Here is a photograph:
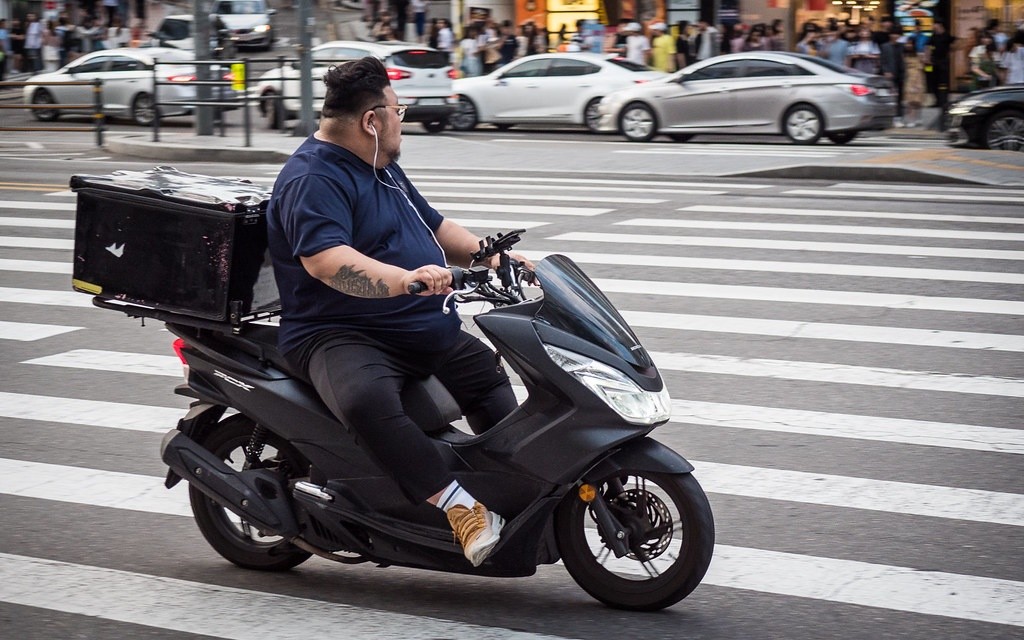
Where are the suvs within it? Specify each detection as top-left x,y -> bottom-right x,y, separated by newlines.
254,39 -> 459,135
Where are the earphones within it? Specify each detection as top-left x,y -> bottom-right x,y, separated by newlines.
369,122 -> 376,132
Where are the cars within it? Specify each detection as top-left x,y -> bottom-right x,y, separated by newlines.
944,82 -> 1024,151
441,50 -> 676,135
144,13 -> 226,58
207,0 -> 279,52
22,47 -> 248,127
597,51 -> 900,146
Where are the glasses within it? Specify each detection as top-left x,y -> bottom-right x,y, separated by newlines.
369,103 -> 408,123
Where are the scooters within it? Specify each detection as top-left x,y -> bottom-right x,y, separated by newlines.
159,227 -> 716,613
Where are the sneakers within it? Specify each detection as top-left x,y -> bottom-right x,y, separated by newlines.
447,501 -> 507,567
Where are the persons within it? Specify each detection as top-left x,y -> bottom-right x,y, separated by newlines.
266,57 -> 540,567
295,0 -> 1024,130
0,0 -> 230,90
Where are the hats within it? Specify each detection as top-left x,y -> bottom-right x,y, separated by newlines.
622,22 -> 642,31
648,22 -> 667,31
